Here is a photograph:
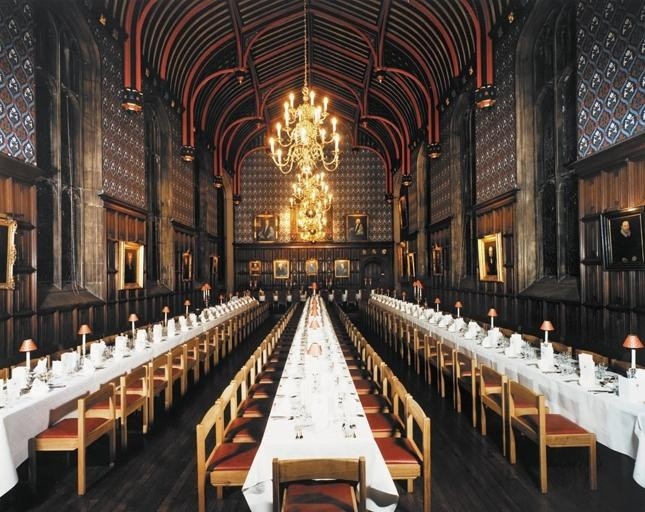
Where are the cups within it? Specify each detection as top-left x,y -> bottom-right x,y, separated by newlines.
476,329 -> 639,397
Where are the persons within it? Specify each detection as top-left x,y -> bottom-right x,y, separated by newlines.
612,220 -> 640,262
351,218 -> 364,240
258,219 -> 274,239
486,246 -> 497,275
126,252 -> 135,283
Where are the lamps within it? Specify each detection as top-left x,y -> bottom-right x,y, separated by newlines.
269,0 -> 343,243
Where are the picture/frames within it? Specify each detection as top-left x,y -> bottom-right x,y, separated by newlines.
406,253 -> 415,278
254,214 -> 278,244
273,260 -> 289,279
599,206 -> 645,271
432,247 -> 444,276
477,232 -> 504,282
334,260 -> 350,278
181,253 -> 191,281
118,240 -> 144,290
346,214 -> 369,242
0,218 -> 18,290
305,259 -> 318,274
249,261 -> 262,276
